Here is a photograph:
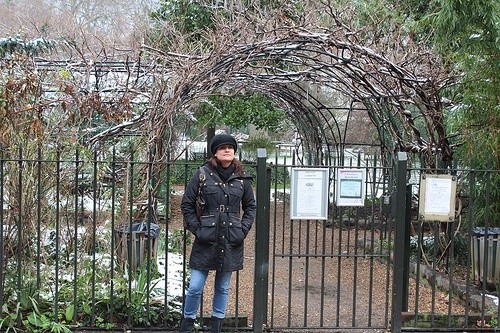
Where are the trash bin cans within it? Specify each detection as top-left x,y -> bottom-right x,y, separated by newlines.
114,220 -> 161,279
470,226 -> 500,293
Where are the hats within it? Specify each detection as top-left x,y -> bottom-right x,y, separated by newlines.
210,133 -> 238,155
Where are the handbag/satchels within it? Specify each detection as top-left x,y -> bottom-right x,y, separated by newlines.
182,165 -> 207,234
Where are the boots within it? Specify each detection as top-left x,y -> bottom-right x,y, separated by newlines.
179,317 -> 196,332
211,315 -> 224,332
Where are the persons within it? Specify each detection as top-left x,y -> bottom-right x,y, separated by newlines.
179,134 -> 256,333
